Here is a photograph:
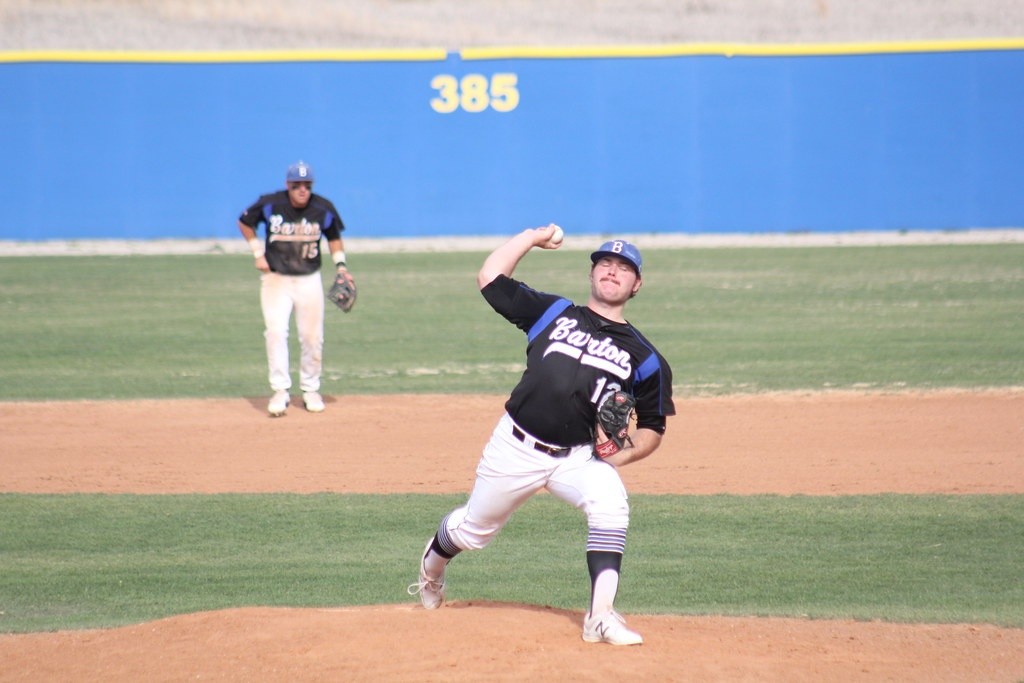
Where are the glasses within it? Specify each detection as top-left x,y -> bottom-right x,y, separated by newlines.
286,185 -> 311,191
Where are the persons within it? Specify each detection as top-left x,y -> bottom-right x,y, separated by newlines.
408,224 -> 677,646
237,161 -> 353,417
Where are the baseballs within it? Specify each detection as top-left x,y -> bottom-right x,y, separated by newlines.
546,223 -> 565,245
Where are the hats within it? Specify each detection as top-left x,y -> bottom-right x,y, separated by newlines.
591,240 -> 642,275
286,163 -> 314,182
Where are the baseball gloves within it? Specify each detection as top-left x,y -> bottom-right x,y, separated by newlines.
591,392 -> 638,452
326,272 -> 356,314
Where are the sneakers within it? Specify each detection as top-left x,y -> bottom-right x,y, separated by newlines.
582,609 -> 643,646
407,537 -> 450,610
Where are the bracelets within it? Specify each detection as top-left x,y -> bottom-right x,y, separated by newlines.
335,262 -> 349,270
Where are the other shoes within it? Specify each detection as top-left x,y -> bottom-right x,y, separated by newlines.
303,391 -> 324,412
267,391 -> 290,417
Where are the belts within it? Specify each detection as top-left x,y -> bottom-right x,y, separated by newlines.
512,425 -> 570,458
